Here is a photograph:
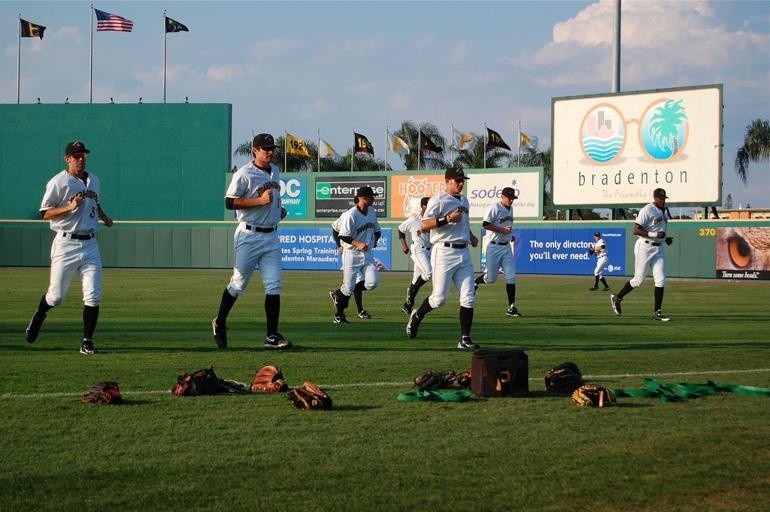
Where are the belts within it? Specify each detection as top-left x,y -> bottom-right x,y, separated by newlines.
246,225 -> 277,234
422,246 -> 430,250
445,242 -> 467,248
646,240 -> 661,246
63,233 -> 94,239
491,241 -> 508,245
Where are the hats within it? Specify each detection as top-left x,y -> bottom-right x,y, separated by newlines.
654,189 -> 669,199
253,134 -> 279,149
502,188 -> 517,200
445,167 -> 470,181
594,232 -> 600,236
65,142 -> 89,155
356,186 -> 379,197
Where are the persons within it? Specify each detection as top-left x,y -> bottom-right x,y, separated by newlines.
26,140 -> 114,357
587,231 -> 609,291
471,186 -> 522,319
608,187 -> 673,323
407,168 -> 480,350
398,196 -> 433,315
331,186 -> 381,323
328,197 -> 381,309
209,134 -> 295,353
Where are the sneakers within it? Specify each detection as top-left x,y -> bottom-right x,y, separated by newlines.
602,288 -> 610,291
611,294 -> 622,315
26,309 -> 46,342
334,315 -> 351,325
357,310 -> 371,320
264,335 -> 292,350
653,309 -> 672,322
329,290 -> 342,312
589,286 -> 599,290
406,309 -> 423,339
506,306 -> 520,318
79,340 -> 98,356
458,336 -> 480,352
406,287 -> 418,308
401,305 -> 413,319
212,315 -> 227,347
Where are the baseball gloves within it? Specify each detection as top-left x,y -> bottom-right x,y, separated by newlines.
545,362 -> 582,395
589,245 -> 594,254
410,369 -> 473,390
571,384 -> 617,406
290,381 -> 331,409
172,368 -> 249,395
80,381 -> 121,404
250,364 -> 288,392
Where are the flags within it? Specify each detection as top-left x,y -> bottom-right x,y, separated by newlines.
487,128 -> 511,152
95,9 -> 133,34
353,134 -> 375,157
453,129 -> 479,156
420,130 -> 445,154
319,138 -> 344,161
386,130 -> 410,158
519,129 -> 539,151
21,19 -> 45,40
284,133 -> 312,158
165,17 -> 189,35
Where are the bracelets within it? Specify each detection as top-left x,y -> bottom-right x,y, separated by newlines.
436,215 -> 448,228
647,231 -> 657,238
447,214 -> 452,223
